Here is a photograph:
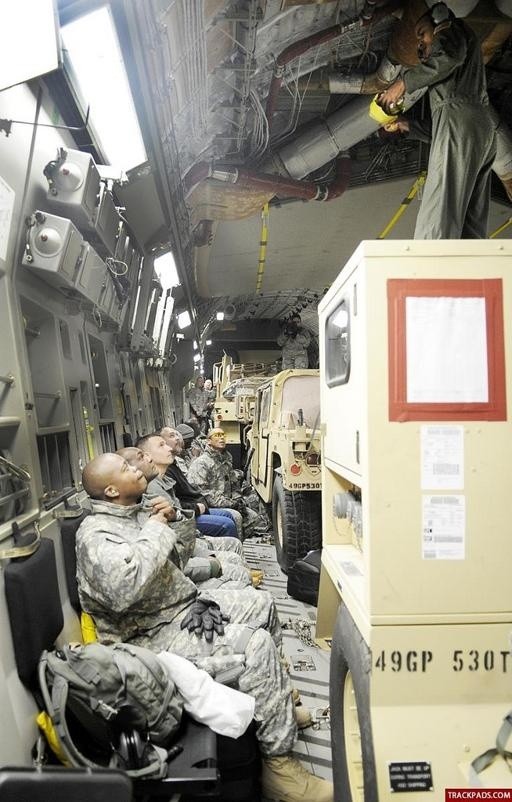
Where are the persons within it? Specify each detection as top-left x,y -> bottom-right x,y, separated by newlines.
378,0 -> 498,240
277,312 -> 310,369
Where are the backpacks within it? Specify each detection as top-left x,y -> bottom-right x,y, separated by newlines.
40,643 -> 184,781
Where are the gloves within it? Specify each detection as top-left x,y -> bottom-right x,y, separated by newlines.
180,599 -> 231,642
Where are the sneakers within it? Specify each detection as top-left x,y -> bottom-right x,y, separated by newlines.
262,690 -> 334,802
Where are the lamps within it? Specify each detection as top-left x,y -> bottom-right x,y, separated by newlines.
55,1 -> 153,185
153,239 -> 181,290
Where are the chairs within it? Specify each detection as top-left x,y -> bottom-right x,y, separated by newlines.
60,509 -> 93,618
1,530 -> 265,801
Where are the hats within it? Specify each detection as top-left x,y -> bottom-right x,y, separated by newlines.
175,424 -> 195,439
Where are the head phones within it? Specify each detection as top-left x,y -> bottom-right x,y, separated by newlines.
430,2 -> 451,25
106,701 -> 151,770
